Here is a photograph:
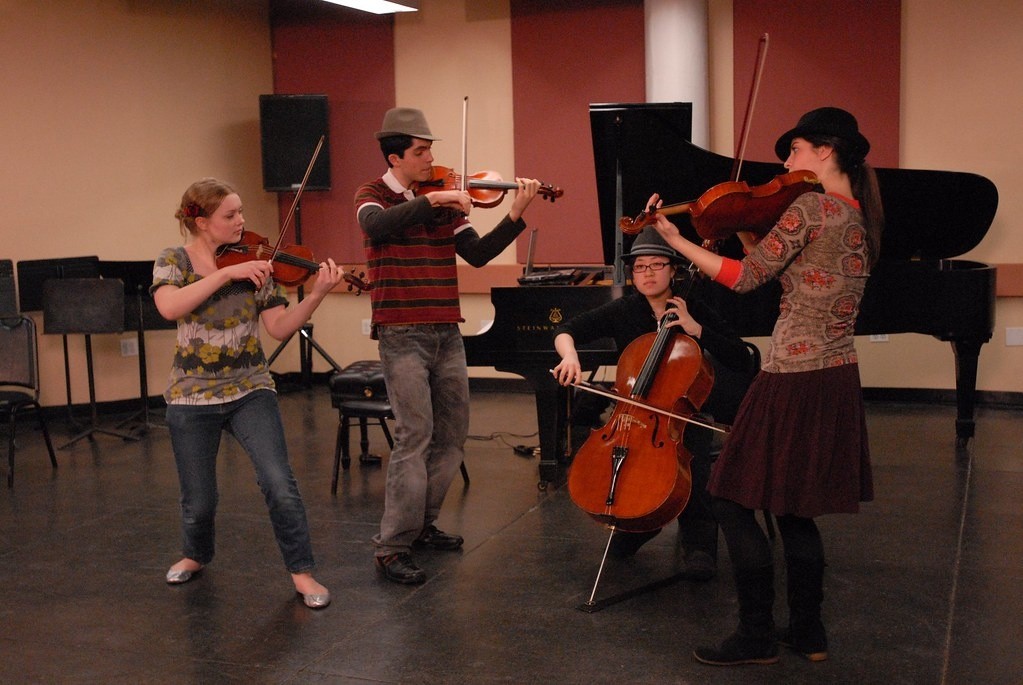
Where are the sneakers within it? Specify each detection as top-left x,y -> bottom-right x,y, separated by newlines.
417,525 -> 465,549
376,552 -> 427,583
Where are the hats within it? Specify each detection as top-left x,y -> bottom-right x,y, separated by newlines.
775,107 -> 870,163
618,227 -> 684,262
375,108 -> 438,142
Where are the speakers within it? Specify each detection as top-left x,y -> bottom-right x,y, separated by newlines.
258,93 -> 332,192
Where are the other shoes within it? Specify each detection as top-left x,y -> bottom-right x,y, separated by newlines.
688,553 -> 718,579
604,529 -> 662,558
296,576 -> 332,609
166,561 -> 206,584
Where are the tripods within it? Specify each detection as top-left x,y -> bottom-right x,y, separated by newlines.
15,254 -> 166,450
266,192 -> 342,388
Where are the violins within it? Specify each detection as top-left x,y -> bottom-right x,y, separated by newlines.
413,162 -> 564,208
618,169 -> 825,239
213,227 -> 374,295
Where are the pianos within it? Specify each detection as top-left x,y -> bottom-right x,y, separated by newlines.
461,102 -> 998,491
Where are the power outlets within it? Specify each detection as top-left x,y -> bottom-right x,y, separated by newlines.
120,338 -> 138,357
870,334 -> 889,342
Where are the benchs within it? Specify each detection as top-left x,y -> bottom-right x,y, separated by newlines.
330,360 -> 470,500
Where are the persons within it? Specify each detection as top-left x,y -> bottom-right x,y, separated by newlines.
148,178 -> 346,609
640,107 -> 884,666
353,107 -> 542,585
550,226 -> 752,582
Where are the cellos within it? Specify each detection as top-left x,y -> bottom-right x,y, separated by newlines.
568,235 -> 720,613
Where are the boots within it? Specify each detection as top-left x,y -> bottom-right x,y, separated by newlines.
775,552 -> 831,660
692,562 -> 781,665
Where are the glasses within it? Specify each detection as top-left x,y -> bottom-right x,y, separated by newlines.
632,261 -> 674,273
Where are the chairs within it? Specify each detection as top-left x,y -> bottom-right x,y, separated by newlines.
708,342 -> 779,545
0,315 -> 58,489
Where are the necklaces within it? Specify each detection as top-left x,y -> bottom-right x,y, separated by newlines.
652,314 -> 662,321
